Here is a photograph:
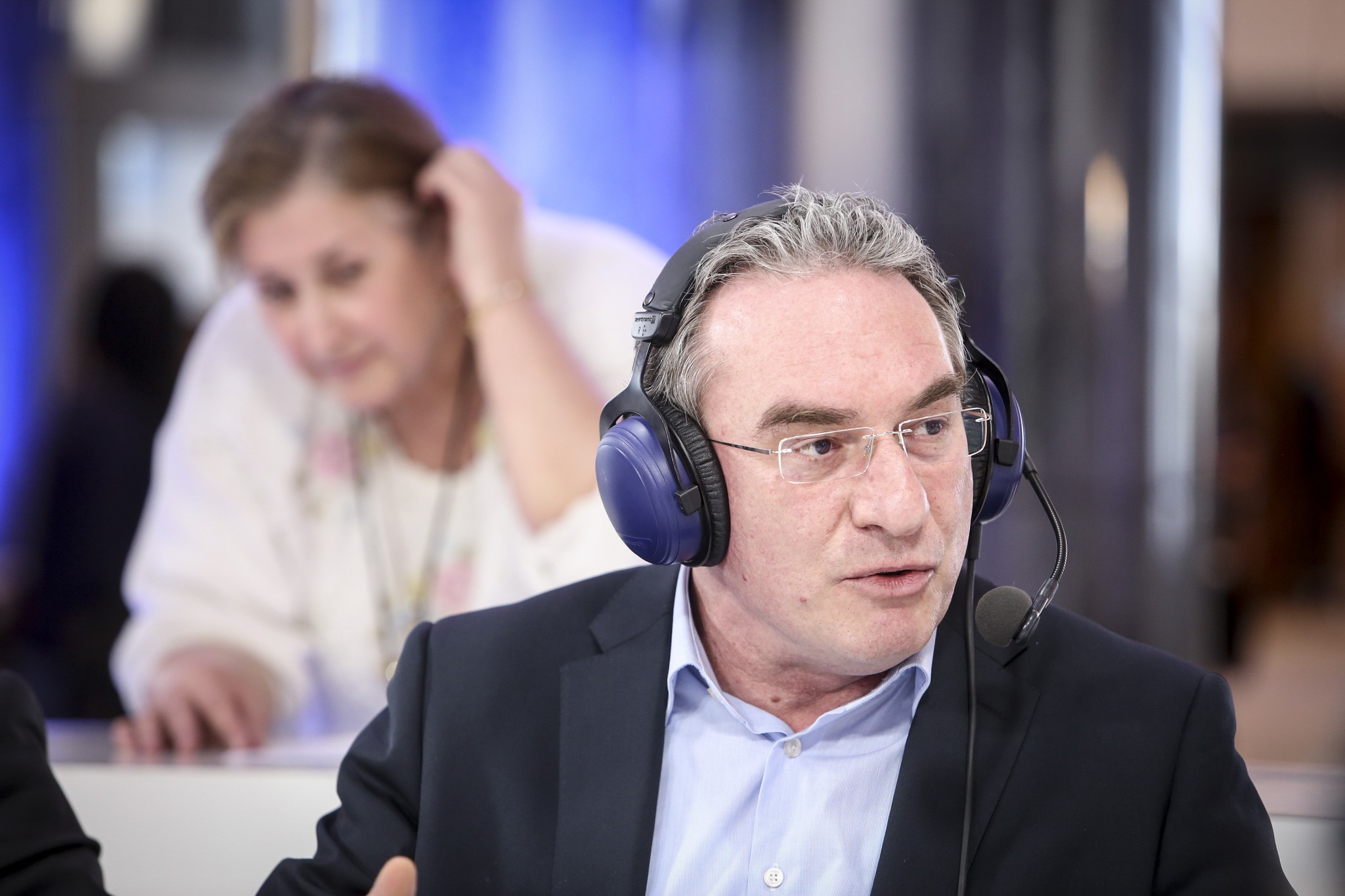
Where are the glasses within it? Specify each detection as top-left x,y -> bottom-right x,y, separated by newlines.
710,405 -> 992,486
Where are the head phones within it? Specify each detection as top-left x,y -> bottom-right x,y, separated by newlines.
595,193 -> 1025,567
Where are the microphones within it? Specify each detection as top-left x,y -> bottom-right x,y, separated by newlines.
974,443 -> 1075,648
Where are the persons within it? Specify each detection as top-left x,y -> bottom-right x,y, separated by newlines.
0,74 -> 673,754
254,185 -> 1300,896
